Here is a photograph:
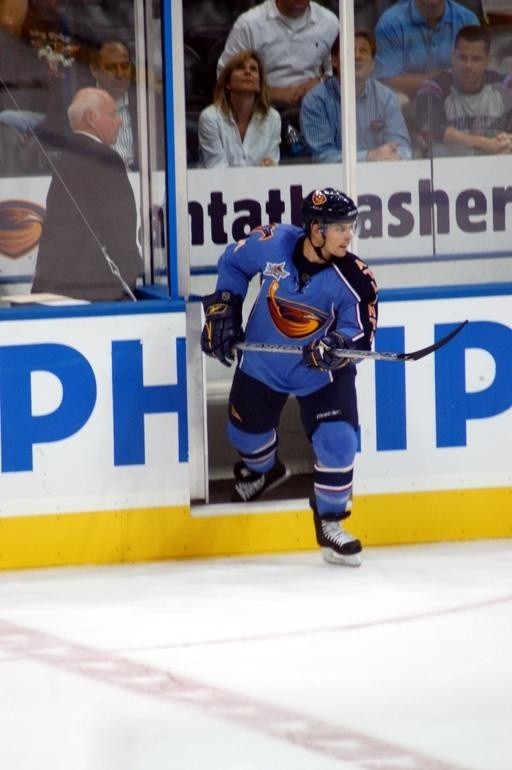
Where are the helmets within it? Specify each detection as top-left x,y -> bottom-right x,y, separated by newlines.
298,187 -> 358,238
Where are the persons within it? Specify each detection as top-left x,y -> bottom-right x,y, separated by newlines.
200,186 -> 377,568
198,49 -> 281,167
217,0 -> 340,109
88,35 -> 138,172
371,0 -> 482,98
405,25 -> 512,156
32,88 -> 142,302
299,29 -> 413,163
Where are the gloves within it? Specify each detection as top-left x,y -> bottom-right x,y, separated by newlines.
303,330 -> 350,373
200,289 -> 244,367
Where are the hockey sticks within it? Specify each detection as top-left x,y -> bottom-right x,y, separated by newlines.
232,319 -> 471,362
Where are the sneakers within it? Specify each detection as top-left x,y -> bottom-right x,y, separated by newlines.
314,509 -> 362,555
231,459 -> 286,503
